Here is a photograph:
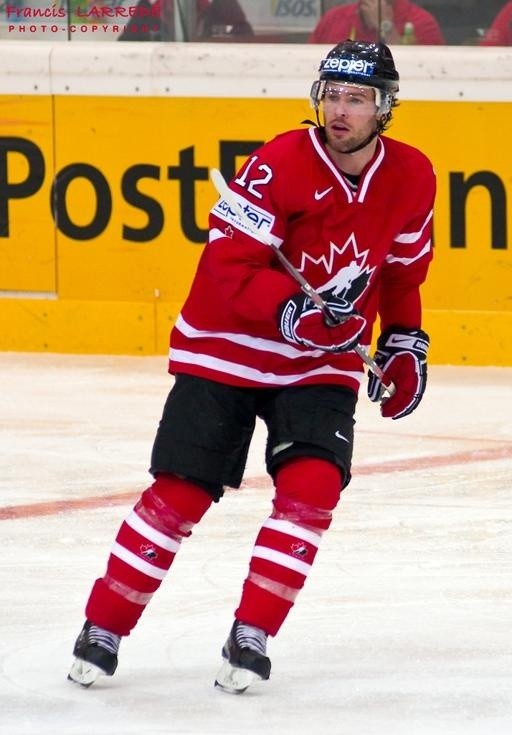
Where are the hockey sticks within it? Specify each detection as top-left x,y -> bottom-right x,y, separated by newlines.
209,168 -> 396,396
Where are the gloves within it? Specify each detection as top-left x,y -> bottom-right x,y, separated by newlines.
276,286 -> 369,354
365,322 -> 431,421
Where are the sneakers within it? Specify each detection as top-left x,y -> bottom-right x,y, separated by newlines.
71,613 -> 122,677
220,616 -> 271,682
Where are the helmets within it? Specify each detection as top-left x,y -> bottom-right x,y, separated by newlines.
316,39 -> 402,108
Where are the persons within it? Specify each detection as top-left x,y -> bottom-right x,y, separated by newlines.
307,0 -> 446,46
72,40 -> 435,679
115,0 -> 254,40
479,0 -> 512,46
